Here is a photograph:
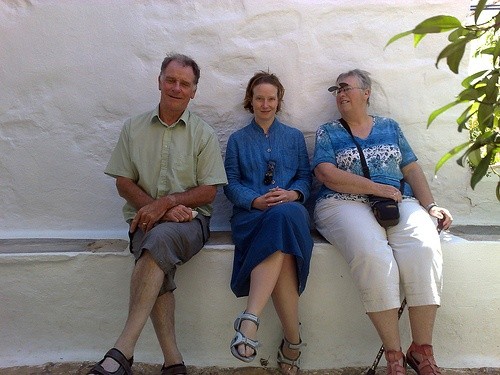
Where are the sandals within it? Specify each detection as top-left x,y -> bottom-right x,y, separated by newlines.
86,347 -> 134,375
277,321 -> 302,375
405,341 -> 442,375
385,346 -> 407,375
230,309 -> 261,363
160,360 -> 188,375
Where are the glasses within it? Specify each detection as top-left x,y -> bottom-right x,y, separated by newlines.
328,81 -> 366,97
263,160 -> 276,185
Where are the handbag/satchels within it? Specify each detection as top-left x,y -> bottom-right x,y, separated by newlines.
372,198 -> 400,227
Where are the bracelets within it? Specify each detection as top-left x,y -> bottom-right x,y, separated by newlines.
425,201 -> 438,211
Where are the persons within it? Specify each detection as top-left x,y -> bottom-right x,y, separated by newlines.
311,69 -> 455,375
223,72 -> 316,375
88,54 -> 229,375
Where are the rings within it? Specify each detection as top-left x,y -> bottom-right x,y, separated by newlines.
394,192 -> 396,196
143,223 -> 147,225
280,201 -> 283,204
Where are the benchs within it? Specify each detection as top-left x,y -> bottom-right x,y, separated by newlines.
0,224 -> 500,367
